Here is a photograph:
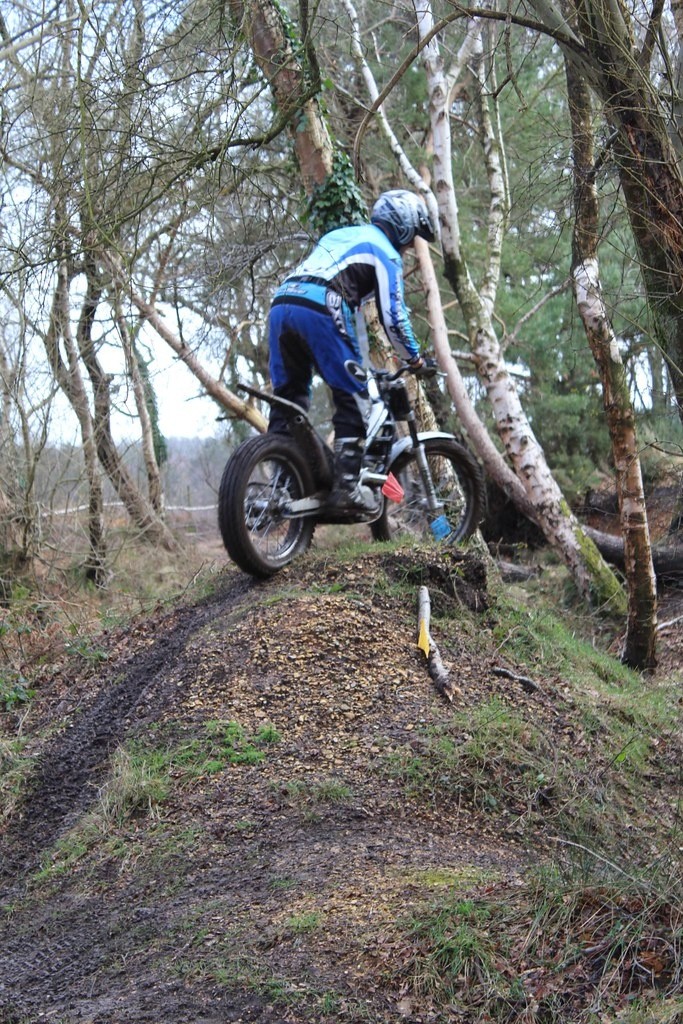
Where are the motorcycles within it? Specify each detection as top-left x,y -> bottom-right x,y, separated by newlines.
217,355 -> 487,580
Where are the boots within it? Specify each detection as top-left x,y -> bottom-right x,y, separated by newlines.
326,436 -> 380,515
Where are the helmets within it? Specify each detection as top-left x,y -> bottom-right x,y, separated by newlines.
371,189 -> 436,252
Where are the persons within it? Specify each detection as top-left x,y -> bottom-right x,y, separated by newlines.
267,189 -> 438,512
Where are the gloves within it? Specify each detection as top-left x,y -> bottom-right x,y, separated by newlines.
413,358 -> 438,371
